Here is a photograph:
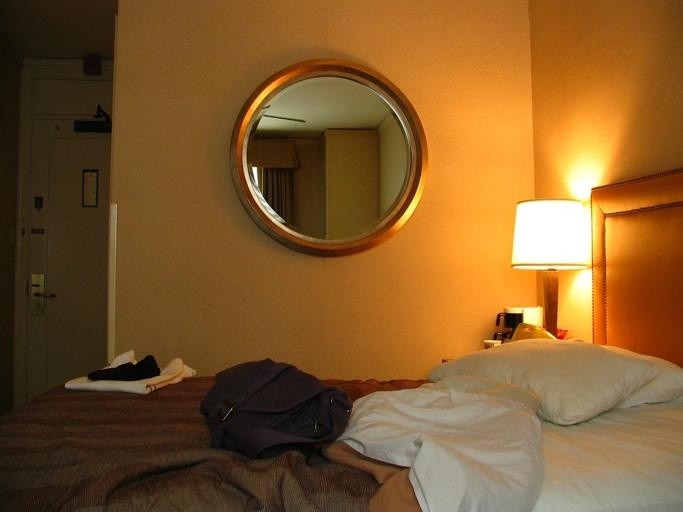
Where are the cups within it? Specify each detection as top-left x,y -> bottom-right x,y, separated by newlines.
482,340 -> 501,349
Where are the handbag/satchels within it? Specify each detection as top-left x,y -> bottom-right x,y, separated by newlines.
200,356 -> 352,463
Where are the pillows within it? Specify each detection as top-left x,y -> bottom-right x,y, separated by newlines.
423,336 -> 682,426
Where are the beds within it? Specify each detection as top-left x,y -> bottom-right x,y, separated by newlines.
0,171 -> 683,512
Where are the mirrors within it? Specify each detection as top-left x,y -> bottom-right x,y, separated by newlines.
229,56 -> 430,257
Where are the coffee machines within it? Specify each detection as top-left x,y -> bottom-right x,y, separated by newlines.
493,307 -> 543,343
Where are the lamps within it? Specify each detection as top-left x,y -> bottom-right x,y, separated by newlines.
510,198 -> 592,341
